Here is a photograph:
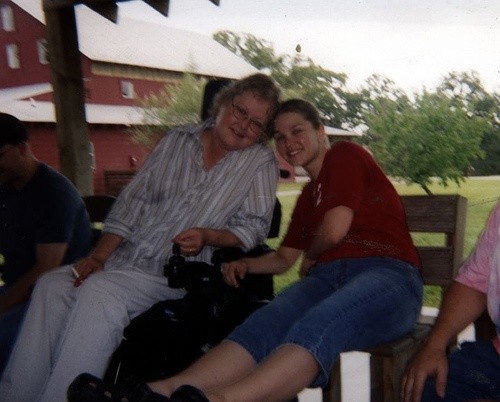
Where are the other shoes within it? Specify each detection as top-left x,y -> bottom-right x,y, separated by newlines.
169,384 -> 209,402
66,372 -> 168,402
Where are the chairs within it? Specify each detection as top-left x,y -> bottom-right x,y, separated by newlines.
103,169 -> 290,399
81,194 -> 119,252
322,194 -> 467,402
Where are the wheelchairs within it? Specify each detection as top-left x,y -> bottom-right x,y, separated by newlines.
100,186 -> 282,383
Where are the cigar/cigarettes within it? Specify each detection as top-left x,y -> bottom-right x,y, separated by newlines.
71,266 -> 84,288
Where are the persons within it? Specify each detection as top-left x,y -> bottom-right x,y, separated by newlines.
399,198 -> 500,402
0,73 -> 278,402
68,98 -> 423,402
0,111 -> 92,381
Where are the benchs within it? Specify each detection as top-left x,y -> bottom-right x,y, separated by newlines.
105,170 -> 138,196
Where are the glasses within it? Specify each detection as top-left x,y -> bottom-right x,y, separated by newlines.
230,96 -> 269,136
